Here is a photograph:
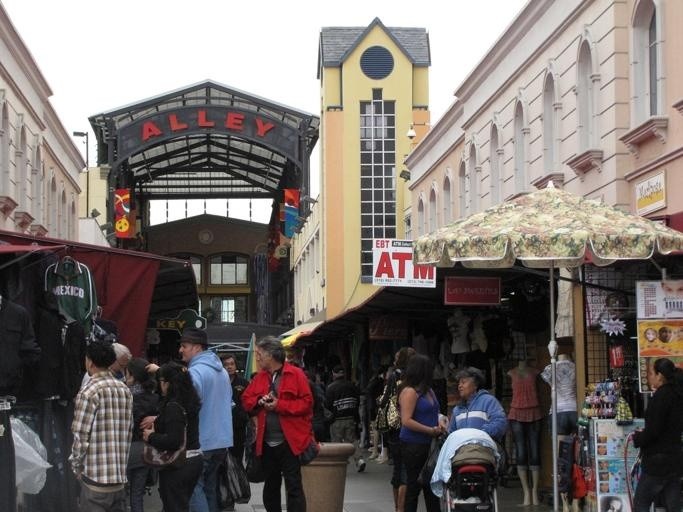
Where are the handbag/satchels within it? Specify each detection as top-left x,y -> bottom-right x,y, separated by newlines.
296,435 -> 321,465
142,399 -> 190,468
371,371 -> 404,435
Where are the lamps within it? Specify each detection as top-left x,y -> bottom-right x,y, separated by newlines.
406,121 -> 432,139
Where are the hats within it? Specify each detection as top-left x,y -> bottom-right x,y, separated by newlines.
173,324 -> 214,348
81,338 -> 118,369
331,363 -> 345,373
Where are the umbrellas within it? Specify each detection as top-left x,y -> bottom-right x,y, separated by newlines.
410,181 -> 683,512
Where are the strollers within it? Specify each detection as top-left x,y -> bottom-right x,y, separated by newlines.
429,425 -> 499,512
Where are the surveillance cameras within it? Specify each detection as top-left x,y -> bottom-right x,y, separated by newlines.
407,128 -> 416,140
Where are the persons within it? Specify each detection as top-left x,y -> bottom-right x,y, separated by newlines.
659,327 -> 673,343
633,359 -> 683,512
540,354 -> 578,436
661,282 -> 683,298
508,359 -> 542,505
645,328 -> 657,341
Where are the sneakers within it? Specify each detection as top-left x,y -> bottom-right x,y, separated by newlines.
354,457 -> 368,473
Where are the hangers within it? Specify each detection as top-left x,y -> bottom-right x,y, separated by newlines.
59,246 -> 73,266
